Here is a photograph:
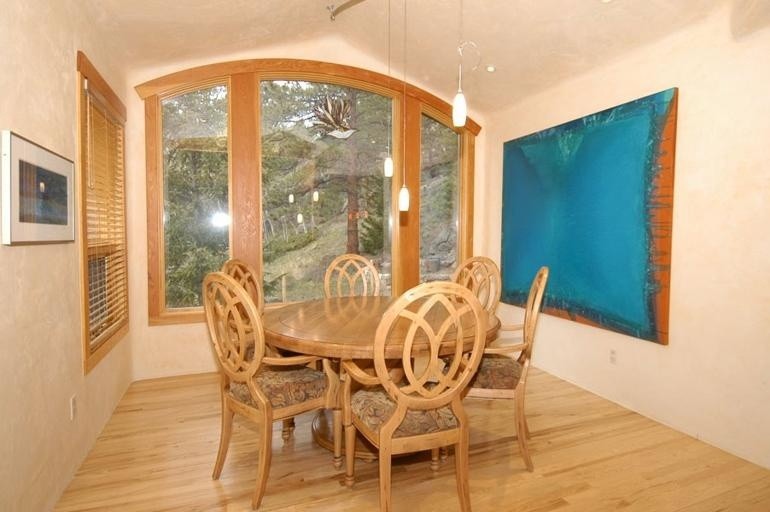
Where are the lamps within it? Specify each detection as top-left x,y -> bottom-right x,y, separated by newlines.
384,2 -> 469,212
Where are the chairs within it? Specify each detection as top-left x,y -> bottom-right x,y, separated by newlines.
201,274 -> 344,503
452,257 -> 503,310
481,263 -> 547,484
320,258 -> 382,304
348,280 -> 477,511
221,259 -> 265,319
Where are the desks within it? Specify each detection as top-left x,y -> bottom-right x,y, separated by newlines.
253,291 -> 509,486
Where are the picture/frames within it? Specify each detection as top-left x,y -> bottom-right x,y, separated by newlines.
2,129 -> 76,247
